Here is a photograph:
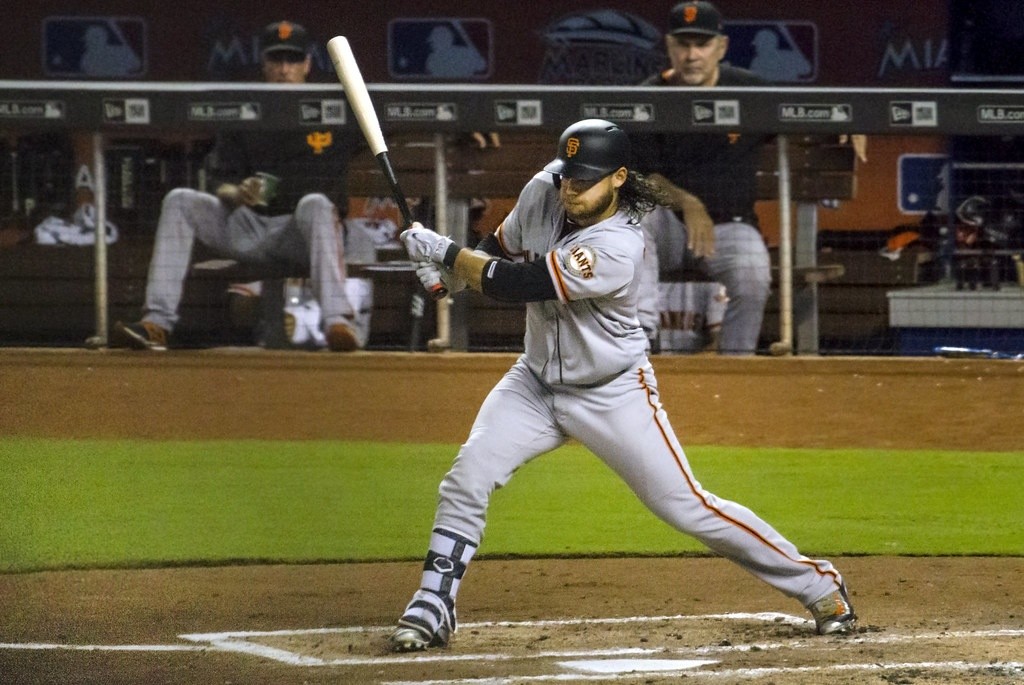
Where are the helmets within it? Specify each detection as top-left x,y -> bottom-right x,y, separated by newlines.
543,118 -> 631,180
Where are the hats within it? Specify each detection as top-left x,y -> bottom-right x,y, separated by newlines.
258,21 -> 309,58
665,2 -> 721,37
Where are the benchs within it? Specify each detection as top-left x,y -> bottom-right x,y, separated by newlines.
199,116 -> 862,356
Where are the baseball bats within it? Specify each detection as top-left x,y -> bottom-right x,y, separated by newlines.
325,33 -> 450,301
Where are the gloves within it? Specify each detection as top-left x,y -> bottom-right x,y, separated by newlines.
399,222 -> 457,267
412,262 -> 442,293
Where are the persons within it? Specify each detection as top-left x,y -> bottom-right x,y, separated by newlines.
381,115 -> 861,653
606,3 -> 773,354
114,26 -> 370,350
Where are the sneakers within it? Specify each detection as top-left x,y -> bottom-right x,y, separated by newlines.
114,321 -> 167,352
391,625 -> 430,651
810,577 -> 857,635
323,314 -> 359,352
